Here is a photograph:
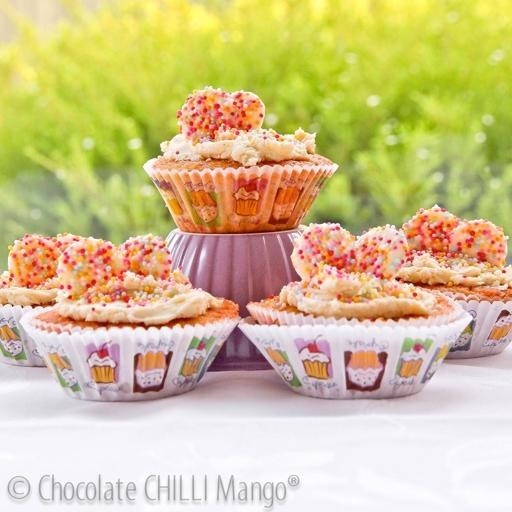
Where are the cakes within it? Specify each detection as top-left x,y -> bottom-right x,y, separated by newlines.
0,232 -> 84,367
237,220 -> 475,400
19,232 -> 241,403
394,203 -> 512,360
141,85 -> 339,231
